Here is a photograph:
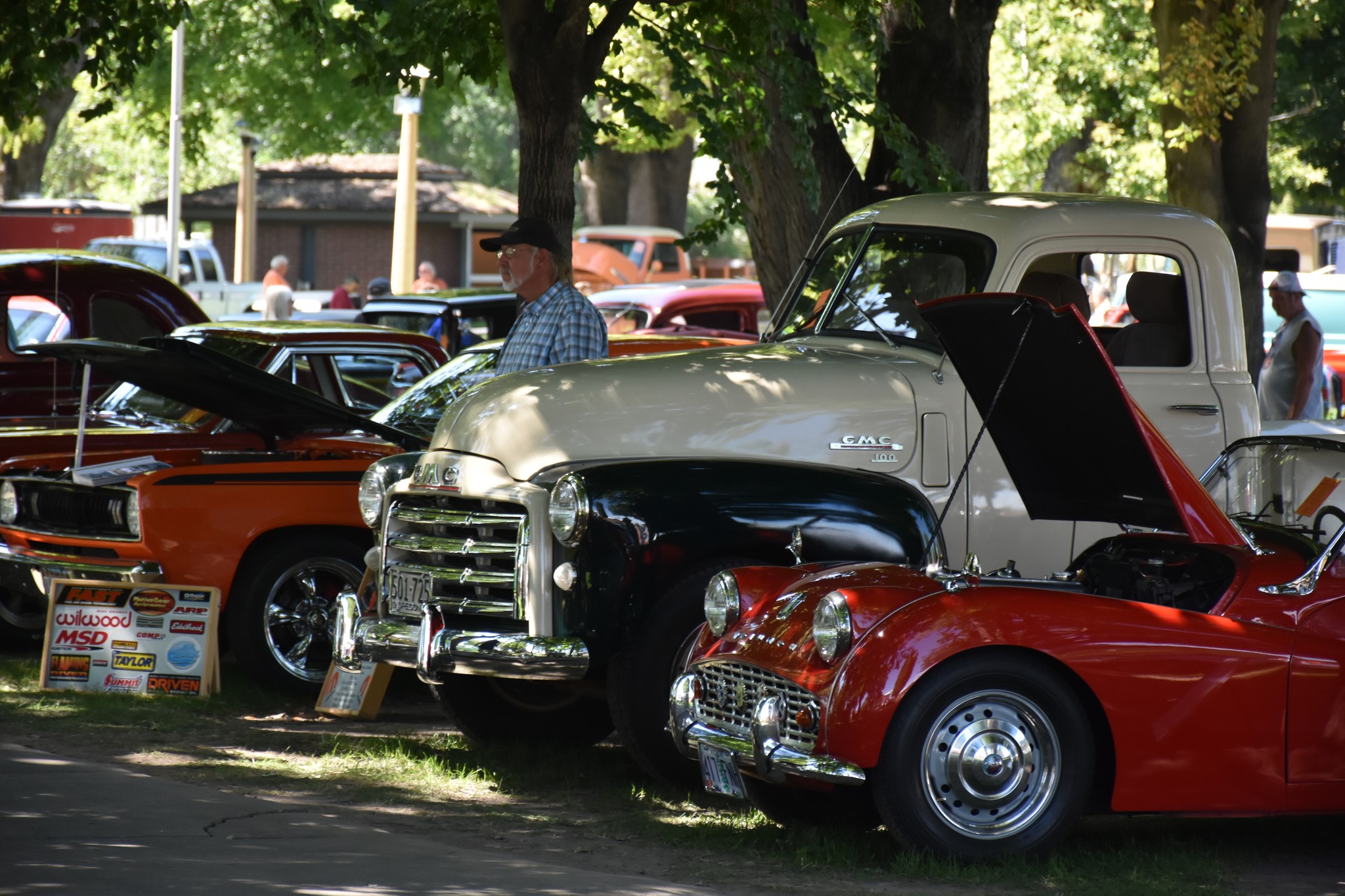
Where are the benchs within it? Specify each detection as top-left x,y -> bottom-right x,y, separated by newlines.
1090,326 -> 1123,351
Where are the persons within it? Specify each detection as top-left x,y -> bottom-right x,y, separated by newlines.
411,261 -> 446,293
329,275 -> 360,308
478,217 -> 608,376
1257,270 -> 1325,524
261,253 -> 300,324
1088,281 -> 1111,328
367,276 -> 393,296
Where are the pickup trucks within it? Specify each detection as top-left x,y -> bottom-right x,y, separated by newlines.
349,193 -> 1345,773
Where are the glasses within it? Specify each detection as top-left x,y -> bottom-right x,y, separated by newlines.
496,246 -> 530,259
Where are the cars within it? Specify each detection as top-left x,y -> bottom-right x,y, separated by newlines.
661,290 -> 1345,875
1091,270 -> 1345,414
0,227 -> 828,716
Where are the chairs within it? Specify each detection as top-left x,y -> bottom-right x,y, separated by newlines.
1106,270 -> 1188,367
1020,270 -> 1091,323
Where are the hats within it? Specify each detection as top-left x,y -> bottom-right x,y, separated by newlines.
477,217 -> 561,255
1267,272 -> 1307,296
368,276 -> 391,295
